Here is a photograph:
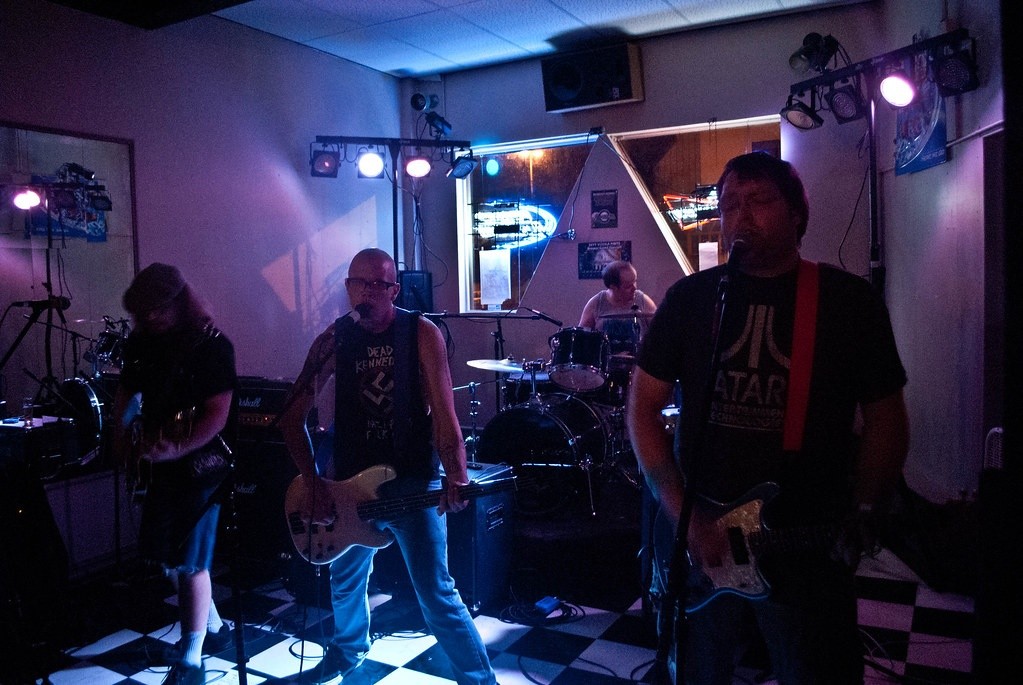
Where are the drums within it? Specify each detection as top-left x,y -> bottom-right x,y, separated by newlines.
549,324 -> 610,392
95,330 -> 128,370
44,375 -> 138,473
616,402 -> 678,460
481,392 -> 614,512
502,368 -> 554,406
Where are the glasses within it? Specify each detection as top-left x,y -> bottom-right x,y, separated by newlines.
711,185 -> 787,219
345,277 -> 396,299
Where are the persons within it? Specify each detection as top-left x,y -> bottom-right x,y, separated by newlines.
578,260 -> 658,342
106,262 -> 236,685
625,150 -> 911,685
279,248 -> 500,685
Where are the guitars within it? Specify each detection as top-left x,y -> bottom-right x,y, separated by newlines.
644,478 -> 897,617
284,463 -> 551,565
125,404 -> 199,505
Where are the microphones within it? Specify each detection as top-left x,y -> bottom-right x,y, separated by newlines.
12,296 -> 71,311
527,307 -> 563,328
322,304 -> 369,342
718,230 -> 754,293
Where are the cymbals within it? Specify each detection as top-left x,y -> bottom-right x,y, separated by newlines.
466,358 -> 527,372
599,311 -> 658,320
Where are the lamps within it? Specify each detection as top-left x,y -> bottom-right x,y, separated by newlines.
780,31 -> 981,134
306,88 -> 479,182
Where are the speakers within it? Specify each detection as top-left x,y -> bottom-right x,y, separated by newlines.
220,434 -> 300,560
44,465 -> 146,578
391,462 -> 518,615
540,42 -> 644,114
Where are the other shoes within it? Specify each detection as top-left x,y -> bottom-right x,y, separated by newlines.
201,621 -> 229,652
308,641 -> 353,684
163,660 -> 205,685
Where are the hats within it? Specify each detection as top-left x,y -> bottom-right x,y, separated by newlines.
122,262 -> 184,315
